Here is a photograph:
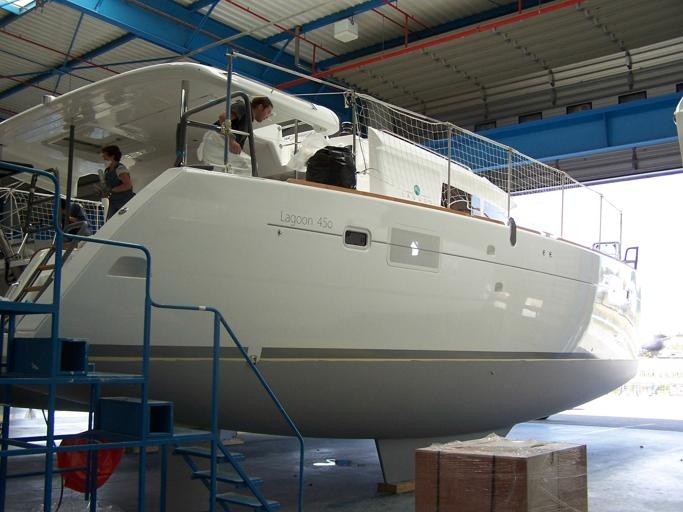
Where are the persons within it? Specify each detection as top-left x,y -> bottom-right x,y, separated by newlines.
49,199 -> 91,249
91,145 -> 136,225
196,94 -> 273,178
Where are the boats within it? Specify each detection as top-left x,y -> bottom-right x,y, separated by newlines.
3,47 -> 652,492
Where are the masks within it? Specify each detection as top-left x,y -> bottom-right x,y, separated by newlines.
103,159 -> 112,169
